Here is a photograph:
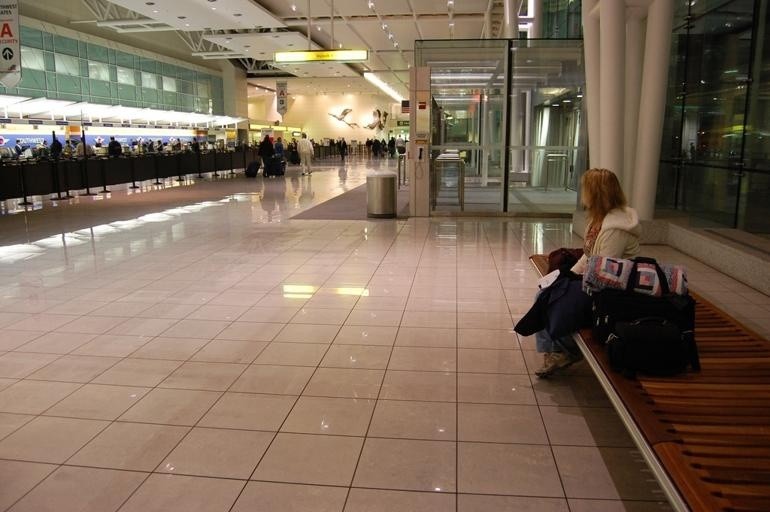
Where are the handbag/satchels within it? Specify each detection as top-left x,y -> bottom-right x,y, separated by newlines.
612,314 -> 697,377
591,257 -> 697,351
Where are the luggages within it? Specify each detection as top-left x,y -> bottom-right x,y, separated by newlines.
245,156 -> 262,177
268,157 -> 286,176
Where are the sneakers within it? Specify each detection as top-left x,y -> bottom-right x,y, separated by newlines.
534,345 -> 582,377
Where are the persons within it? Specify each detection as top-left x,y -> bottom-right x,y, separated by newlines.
257,132 -> 324,178
15,136 -> 254,160
365,134 -> 404,159
338,138 -> 348,160
533,166 -> 645,380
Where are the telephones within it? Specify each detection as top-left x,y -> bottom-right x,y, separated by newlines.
417,147 -> 424,162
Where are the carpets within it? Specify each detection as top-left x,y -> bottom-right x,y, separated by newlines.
288,182 -> 410,220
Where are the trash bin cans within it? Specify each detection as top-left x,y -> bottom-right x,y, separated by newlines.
366,175 -> 397,218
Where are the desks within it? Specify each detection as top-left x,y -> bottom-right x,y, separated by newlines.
432,149 -> 464,210
545,153 -> 568,192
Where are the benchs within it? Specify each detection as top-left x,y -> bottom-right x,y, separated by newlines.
529,254 -> 770,512
